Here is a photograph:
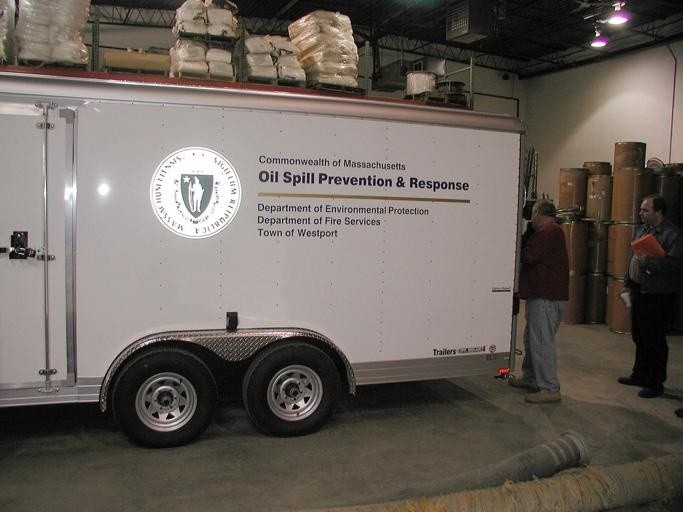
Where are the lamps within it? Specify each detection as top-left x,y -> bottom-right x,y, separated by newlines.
589,2 -> 629,48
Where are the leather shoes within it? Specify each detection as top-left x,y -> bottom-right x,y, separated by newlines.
618,377 -> 664,397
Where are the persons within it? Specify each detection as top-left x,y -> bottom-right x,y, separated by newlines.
507,198 -> 569,405
618,193 -> 681,397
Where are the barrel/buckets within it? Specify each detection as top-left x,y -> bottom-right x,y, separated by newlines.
559,142 -> 683,334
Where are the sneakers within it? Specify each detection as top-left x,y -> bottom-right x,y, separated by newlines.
526,391 -> 561,403
509,378 -> 538,389
